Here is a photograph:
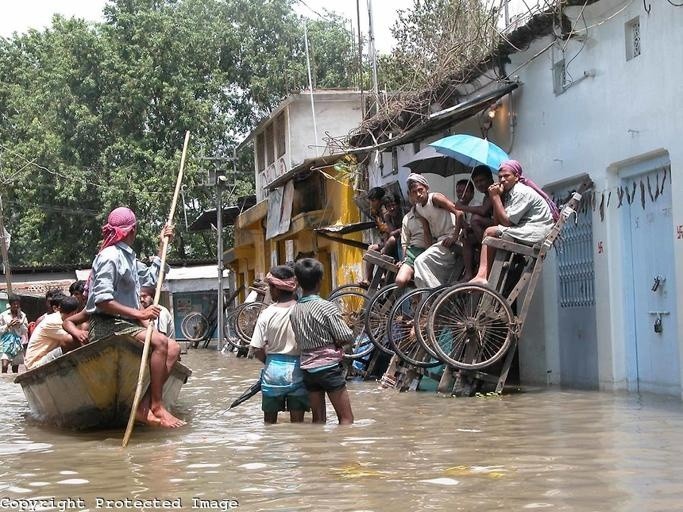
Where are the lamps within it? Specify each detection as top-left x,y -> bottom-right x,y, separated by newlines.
489,103 -> 498,118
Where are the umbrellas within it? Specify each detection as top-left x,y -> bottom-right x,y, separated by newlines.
222,378 -> 260,416
427,134 -> 511,204
401,146 -> 474,206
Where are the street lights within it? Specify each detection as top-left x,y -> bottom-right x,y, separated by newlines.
216,175 -> 229,352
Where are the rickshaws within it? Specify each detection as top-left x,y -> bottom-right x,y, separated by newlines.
181,286 -> 244,349
220,281 -> 270,359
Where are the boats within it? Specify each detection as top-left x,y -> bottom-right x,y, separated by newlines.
14,331 -> 194,431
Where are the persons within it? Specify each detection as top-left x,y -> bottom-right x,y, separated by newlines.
289,259 -> 357,425
363,161 -> 558,342
0,207 -> 188,429
248,265 -> 312,424
